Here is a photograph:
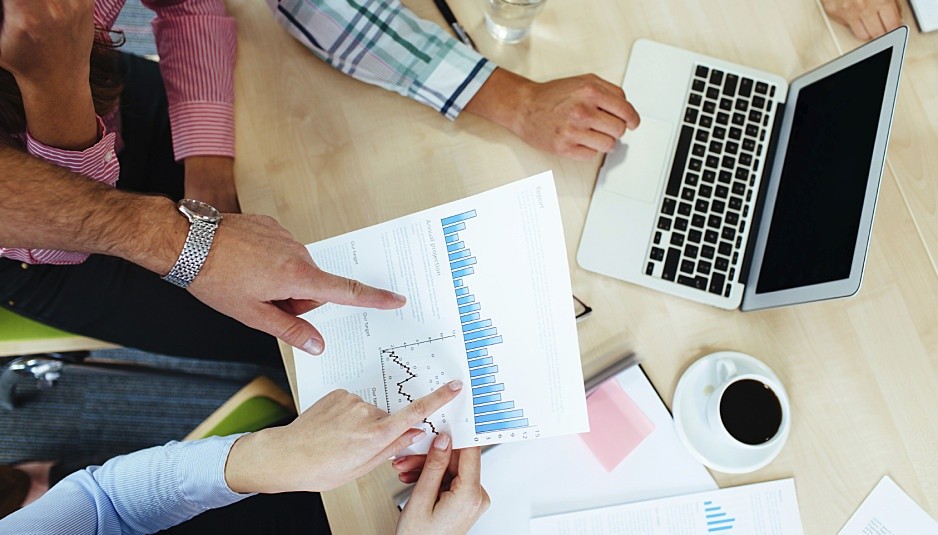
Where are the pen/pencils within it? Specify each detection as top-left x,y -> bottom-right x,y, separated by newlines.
433,0 -> 478,52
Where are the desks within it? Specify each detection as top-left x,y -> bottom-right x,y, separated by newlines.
232,0 -> 938,535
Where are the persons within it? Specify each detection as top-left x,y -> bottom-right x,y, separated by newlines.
0,0 -> 905,534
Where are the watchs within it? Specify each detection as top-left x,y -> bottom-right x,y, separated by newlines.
160,196 -> 224,291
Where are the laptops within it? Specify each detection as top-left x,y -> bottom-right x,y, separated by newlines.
576,23 -> 908,312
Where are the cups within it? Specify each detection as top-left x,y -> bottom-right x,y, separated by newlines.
706,374 -> 791,450
485,0 -> 547,43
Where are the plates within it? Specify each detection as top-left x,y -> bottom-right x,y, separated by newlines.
672,351 -> 790,474
